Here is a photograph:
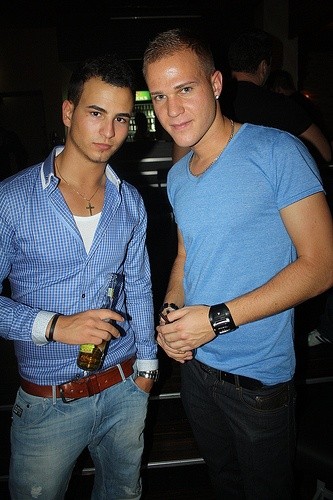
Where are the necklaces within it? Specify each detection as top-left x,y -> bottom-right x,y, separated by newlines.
188,119 -> 234,176
54,156 -> 103,215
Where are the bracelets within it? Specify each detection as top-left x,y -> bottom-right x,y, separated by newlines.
208,303 -> 238,335
48,313 -> 64,343
160,303 -> 180,311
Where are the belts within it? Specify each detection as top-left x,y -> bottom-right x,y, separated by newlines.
17,356 -> 136,403
194,361 -> 264,393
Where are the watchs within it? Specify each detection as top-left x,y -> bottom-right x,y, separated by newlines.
136,370 -> 158,382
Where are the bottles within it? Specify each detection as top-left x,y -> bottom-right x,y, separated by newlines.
77,273 -> 122,371
129,103 -> 155,131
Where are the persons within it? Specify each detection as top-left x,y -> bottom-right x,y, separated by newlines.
0,49 -> 158,500
140,29 -> 333,500
